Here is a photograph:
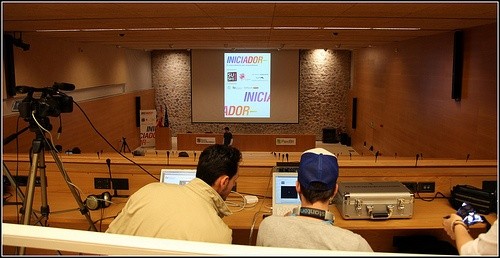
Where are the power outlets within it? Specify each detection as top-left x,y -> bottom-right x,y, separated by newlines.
93,178 -> 129,190
402,181 -> 435,193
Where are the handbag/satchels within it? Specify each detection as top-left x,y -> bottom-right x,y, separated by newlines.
450,184 -> 495,215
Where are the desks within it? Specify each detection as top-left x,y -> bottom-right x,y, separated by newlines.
3,133 -> 497,256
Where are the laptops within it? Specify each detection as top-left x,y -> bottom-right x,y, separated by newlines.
272,172 -> 302,217
159,168 -> 197,185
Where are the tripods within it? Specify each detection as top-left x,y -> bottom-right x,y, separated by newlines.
2,122 -> 98,255
118,138 -> 131,153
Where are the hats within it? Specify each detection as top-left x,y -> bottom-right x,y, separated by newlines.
298,148 -> 339,191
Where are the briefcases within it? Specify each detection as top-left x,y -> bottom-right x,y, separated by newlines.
335,181 -> 414,220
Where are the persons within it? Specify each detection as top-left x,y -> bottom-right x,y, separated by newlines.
256,147 -> 374,253
222,127 -> 233,146
442,214 -> 497,255
105,144 -> 242,245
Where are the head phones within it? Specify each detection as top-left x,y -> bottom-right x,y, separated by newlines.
83,191 -> 112,211
292,207 -> 335,224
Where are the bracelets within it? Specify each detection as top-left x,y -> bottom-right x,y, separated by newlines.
451,220 -> 469,232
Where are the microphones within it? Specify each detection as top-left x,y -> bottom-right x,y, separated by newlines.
466,154 -> 470,162
53,83 -> 75,91
106,159 -> 117,196
334,151 -> 423,167
271,152 -> 288,162
97,150 -> 196,165
